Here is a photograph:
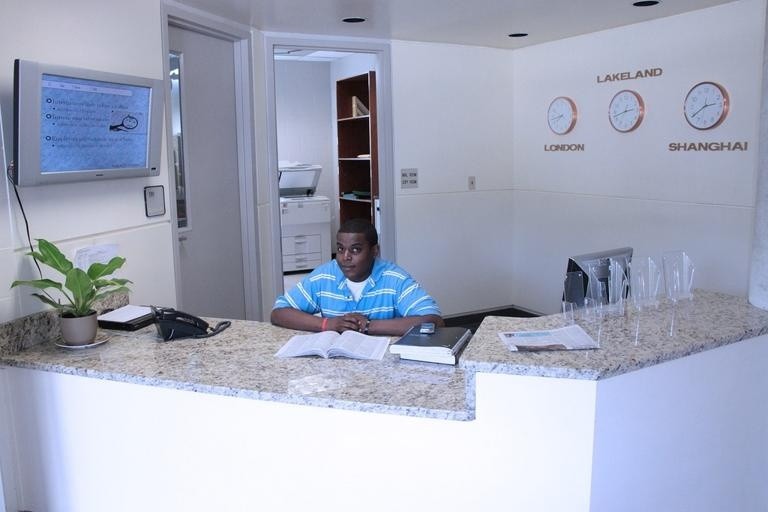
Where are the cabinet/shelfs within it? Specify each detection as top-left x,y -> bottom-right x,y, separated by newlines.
337,69 -> 375,237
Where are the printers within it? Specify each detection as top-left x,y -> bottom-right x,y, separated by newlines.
278,160 -> 333,274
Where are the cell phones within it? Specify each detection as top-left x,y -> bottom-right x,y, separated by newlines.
420,322 -> 435,334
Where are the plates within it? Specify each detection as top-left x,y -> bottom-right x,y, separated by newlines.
54,331 -> 111,349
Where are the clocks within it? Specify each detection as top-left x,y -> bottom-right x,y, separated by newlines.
547,96 -> 577,136
683,81 -> 730,130
608,90 -> 645,133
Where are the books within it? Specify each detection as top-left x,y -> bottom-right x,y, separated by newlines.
389,325 -> 470,356
274,328 -> 391,361
399,333 -> 472,365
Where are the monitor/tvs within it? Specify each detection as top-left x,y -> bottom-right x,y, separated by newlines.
561,247 -> 633,313
12,59 -> 165,187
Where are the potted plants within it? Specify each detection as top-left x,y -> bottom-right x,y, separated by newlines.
8,238 -> 135,349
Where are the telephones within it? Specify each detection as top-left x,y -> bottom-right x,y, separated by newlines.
150,305 -> 209,342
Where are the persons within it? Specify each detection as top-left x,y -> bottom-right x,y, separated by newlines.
270,218 -> 445,335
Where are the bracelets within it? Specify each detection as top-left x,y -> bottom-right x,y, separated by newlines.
322,317 -> 327,330
364,319 -> 370,334
